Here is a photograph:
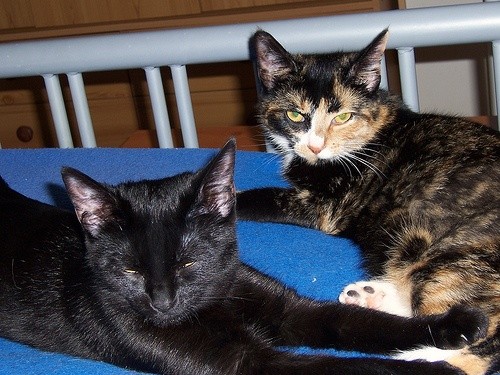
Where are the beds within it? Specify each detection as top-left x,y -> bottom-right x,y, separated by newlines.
0,0 -> 500,375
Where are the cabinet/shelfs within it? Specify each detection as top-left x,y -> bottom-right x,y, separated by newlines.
0,0 -> 408,166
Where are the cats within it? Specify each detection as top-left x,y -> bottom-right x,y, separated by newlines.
235,26 -> 500,375
0,136 -> 490,375
